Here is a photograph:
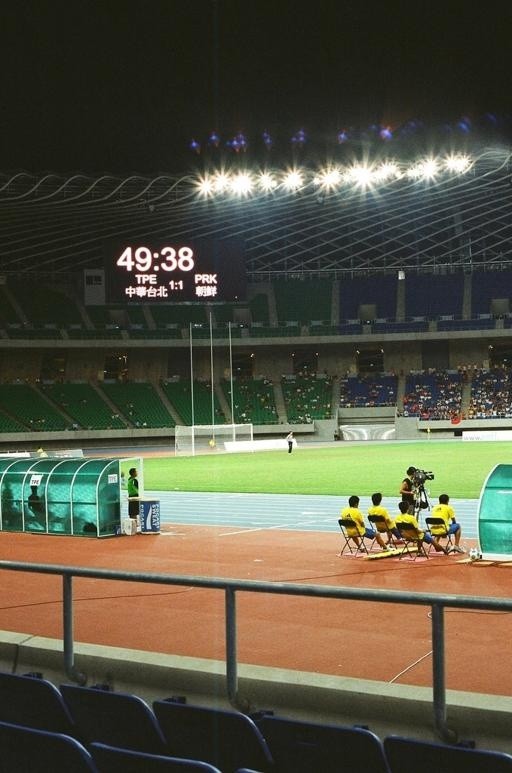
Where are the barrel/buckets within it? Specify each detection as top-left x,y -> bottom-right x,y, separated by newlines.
138,499 -> 161,535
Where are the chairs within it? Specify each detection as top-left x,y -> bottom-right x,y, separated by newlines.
0,670 -> 512,773
395,522 -> 428,561
425,518 -> 455,556
367,515 -> 398,551
0,273 -> 512,433
338,519 -> 369,556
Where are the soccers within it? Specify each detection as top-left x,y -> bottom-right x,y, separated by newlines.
469,551 -> 479,561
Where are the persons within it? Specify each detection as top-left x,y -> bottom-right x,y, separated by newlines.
126,468 -> 139,527
235,362 -> 512,432
340,466 -> 466,554
286,430 -> 293,453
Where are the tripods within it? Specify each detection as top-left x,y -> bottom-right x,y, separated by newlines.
412,485 -> 431,522
